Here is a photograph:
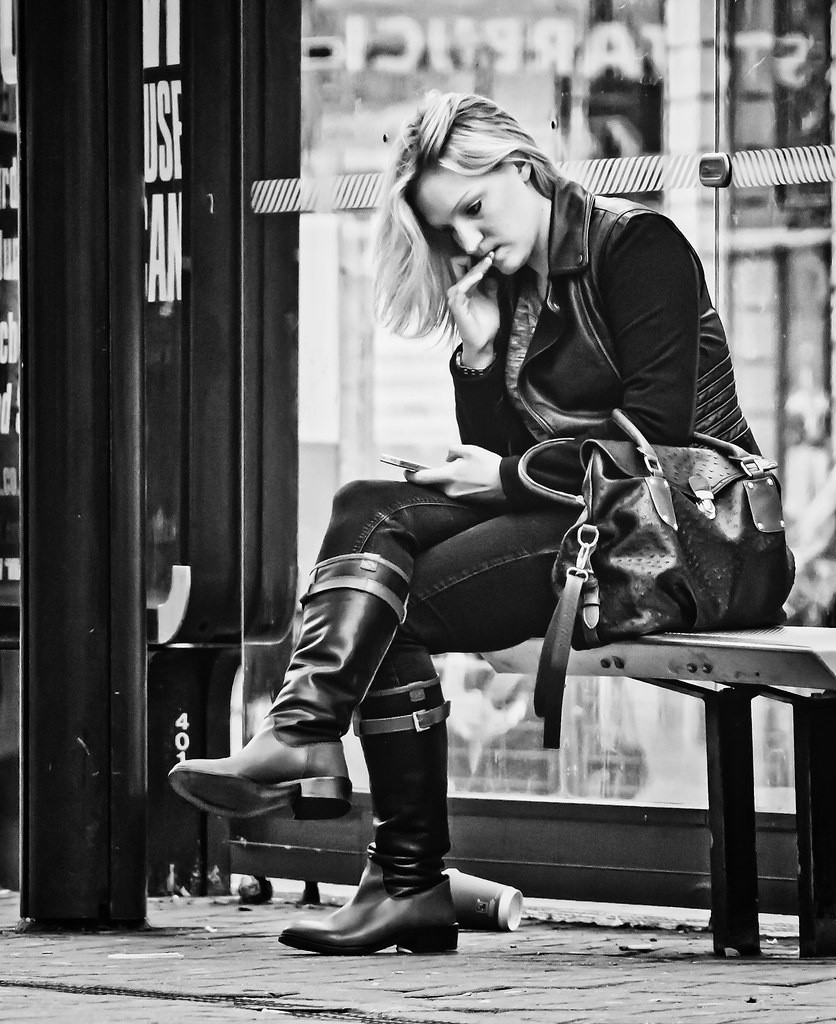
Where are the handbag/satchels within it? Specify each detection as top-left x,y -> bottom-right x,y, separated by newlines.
514,411 -> 796,750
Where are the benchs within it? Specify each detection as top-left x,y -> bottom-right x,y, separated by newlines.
482,622 -> 836,958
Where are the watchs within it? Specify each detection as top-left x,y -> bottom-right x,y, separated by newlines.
455,350 -> 498,376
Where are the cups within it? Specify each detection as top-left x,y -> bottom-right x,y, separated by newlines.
441,868 -> 524,932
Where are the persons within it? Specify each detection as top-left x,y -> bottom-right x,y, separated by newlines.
168,94 -> 764,957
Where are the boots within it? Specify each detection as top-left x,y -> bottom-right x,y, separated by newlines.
167,553 -> 412,816
278,674 -> 459,954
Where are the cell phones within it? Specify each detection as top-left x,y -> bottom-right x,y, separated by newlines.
380,455 -> 433,473
